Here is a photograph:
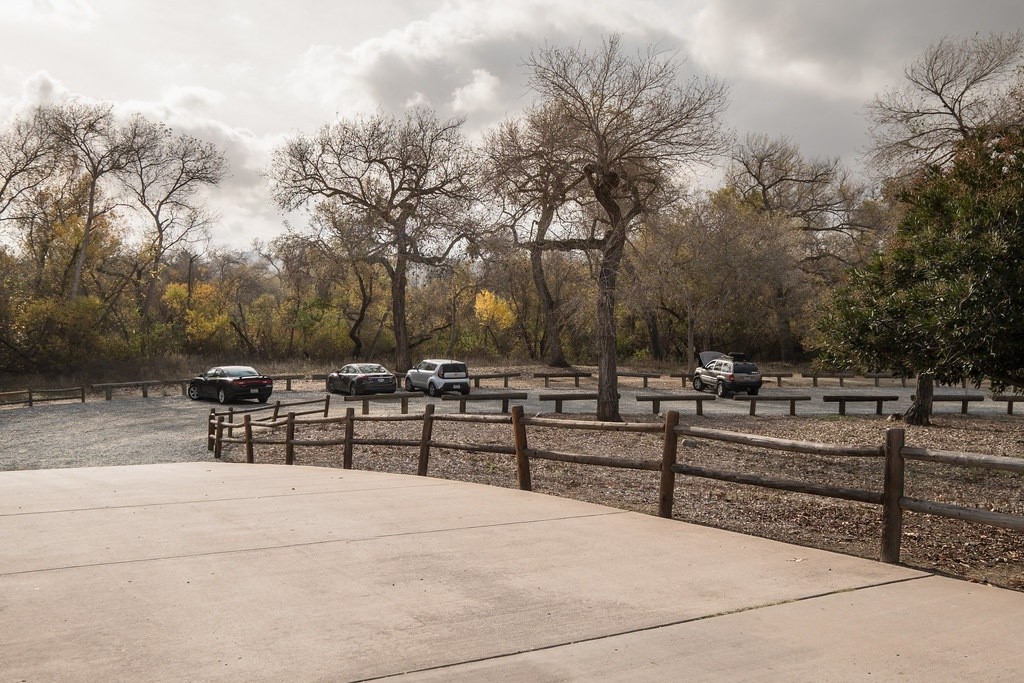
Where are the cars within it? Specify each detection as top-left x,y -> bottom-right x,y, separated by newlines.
188,366 -> 272,404
326,363 -> 396,396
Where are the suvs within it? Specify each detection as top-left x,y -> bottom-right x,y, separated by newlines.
692,351 -> 762,397
405,359 -> 470,397
